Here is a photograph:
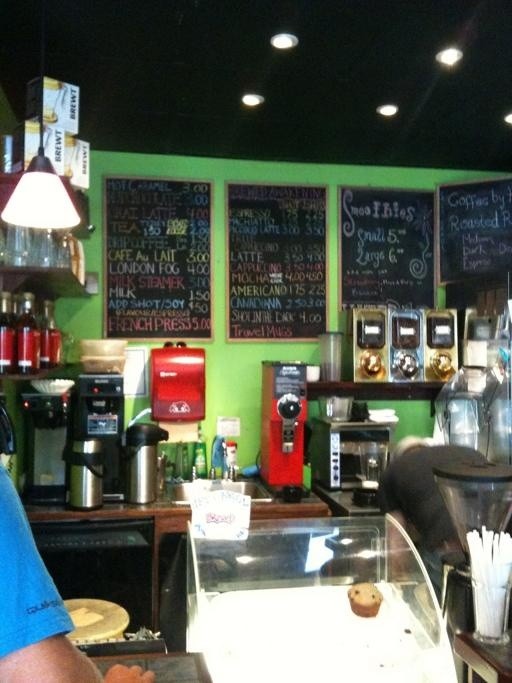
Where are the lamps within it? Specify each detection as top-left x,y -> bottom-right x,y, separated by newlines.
0,0 -> 81,230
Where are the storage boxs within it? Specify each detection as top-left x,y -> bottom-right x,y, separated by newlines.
11,74 -> 91,194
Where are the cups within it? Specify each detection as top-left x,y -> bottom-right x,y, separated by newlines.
1,218 -> 87,285
317,330 -> 343,382
469,579 -> 512,644
352,442 -> 389,489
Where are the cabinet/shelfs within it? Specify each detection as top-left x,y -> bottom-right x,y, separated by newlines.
2,169 -> 99,383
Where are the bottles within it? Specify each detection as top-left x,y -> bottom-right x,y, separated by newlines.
126,423 -> 169,505
0,290 -> 63,375
175,427 -> 239,480
69,435 -> 104,511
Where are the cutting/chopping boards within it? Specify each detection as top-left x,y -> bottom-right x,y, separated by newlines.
60,597 -> 130,642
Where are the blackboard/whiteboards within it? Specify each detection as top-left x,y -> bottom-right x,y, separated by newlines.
336,182 -> 440,311
101,172 -> 215,343
223,180 -> 332,344
436,176 -> 511,284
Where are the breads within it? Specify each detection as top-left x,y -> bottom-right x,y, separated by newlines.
347,582 -> 383,617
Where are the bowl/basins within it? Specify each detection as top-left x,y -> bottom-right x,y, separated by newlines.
317,394 -> 354,421
31,378 -> 75,394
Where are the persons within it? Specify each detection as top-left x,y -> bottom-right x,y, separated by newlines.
370,432 -> 494,612
0,459 -> 157,682
